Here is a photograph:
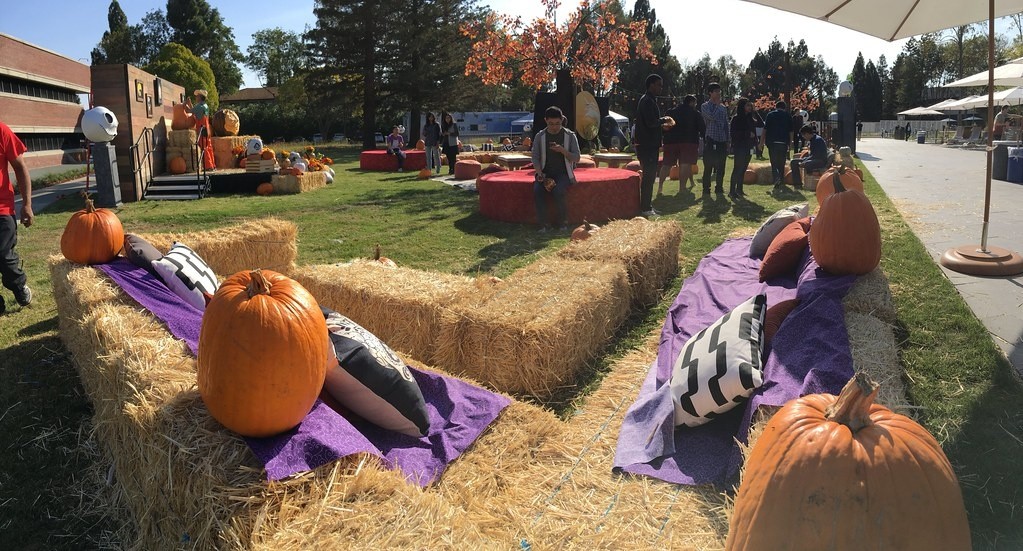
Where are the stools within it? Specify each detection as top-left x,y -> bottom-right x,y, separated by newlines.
456,159 -> 481,180
656,156 -> 664,177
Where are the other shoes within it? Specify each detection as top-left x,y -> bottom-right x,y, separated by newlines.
559,223 -> 567,230
537,224 -> 554,234
436,167 -> 441,173
653,208 -> 661,214
401,152 -> 406,159
399,168 -> 403,172
728,193 -> 738,198
16,285 -> 31,306
737,190 -> 747,196
642,210 -> 660,217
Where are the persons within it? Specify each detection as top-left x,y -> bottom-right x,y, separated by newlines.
756,101 -> 792,188
905,122 -> 911,141
422,112 -> 459,175
183,89 -> 217,171
792,106 -> 805,154
790,124 -> 828,190
631,96 -> 706,194
0,122 -> 35,316
856,120 -> 863,141
728,98 -> 764,197
532,107 -> 580,234
992,104 -> 1014,140
634,74 -> 672,216
387,125 -> 406,173
701,83 -> 730,194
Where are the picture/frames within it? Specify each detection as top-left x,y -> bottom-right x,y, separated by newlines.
135,77 -> 163,118
180,93 -> 185,103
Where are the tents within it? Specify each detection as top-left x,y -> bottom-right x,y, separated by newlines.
511,109 -> 629,142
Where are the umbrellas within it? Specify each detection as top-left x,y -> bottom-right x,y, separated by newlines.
745,0 -> 1023,253
941,118 -> 957,127
963,117 -> 983,126
898,106 -> 944,131
924,97 -> 966,143
939,86 -> 1023,126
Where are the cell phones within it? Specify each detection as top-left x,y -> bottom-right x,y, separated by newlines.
549,142 -> 556,147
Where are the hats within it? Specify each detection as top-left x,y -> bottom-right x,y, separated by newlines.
194,89 -> 207,97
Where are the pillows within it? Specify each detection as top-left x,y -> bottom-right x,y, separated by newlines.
319,305 -> 433,438
762,298 -> 803,364
123,232 -> 165,286
158,241 -> 222,309
672,293 -> 769,434
758,218 -> 812,282
750,203 -> 809,258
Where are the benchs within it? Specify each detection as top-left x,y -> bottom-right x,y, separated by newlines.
478,168 -> 641,226
361,149 -> 428,172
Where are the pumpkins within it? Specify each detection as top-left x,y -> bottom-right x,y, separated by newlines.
239,136 -> 335,195
368,243 -> 398,268
198,267 -> 328,438
60,199 -> 124,264
599,146 -> 808,185
419,166 -> 431,178
571,219 -> 600,240
170,156 -> 186,174
459,136 -> 531,171
416,139 -> 426,150
724,373 -> 975,550
810,162 -> 882,275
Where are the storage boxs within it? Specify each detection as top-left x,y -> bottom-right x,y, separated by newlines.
245,160 -> 273,172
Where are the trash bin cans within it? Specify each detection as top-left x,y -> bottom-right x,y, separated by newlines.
917,131 -> 925,144
1007,147 -> 1023,185
895,126 -> 905,140
992,141 -> 1023,181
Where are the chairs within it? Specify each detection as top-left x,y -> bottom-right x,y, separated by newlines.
948,127 -> 981,146
804,150 -> 834,190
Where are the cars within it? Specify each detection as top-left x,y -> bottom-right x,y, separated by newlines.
332,133 -> 345,142
373,132 -> 384,143
313,133 -> 323,143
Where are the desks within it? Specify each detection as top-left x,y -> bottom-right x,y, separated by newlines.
499,154 -> 532,171
596,152 -> 632,169
